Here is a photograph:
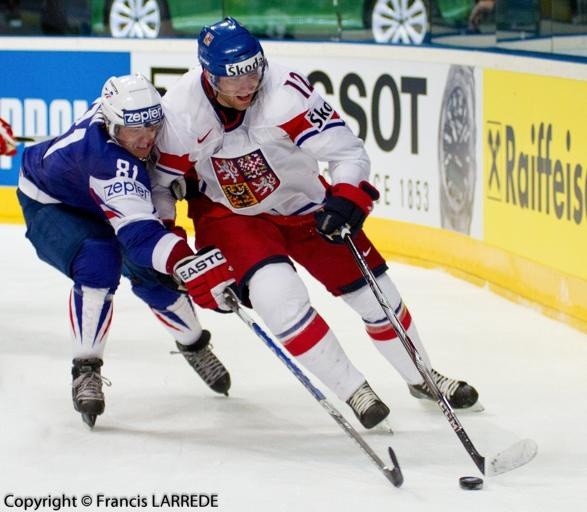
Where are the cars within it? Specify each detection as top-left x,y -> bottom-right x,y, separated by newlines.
47,1 -> 541,46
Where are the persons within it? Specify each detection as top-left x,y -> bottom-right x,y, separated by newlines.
151,15 -> 479,430
16,71 -> 230,415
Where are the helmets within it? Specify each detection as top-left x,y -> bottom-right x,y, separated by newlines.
102,73 -> 164,127
197,16 -> 270,97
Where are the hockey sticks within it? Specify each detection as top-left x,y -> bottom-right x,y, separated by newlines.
339,223 -> 538,476
223,286 -> 403,487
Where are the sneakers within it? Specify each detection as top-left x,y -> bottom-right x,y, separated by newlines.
170,330 -> 230,392
408,369 -> 478,408
346,381 -> 390,428
72,358 -> 111,414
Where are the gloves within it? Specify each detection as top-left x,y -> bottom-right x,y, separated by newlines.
166,242 -> 236,311
315,175 -> 378,244
